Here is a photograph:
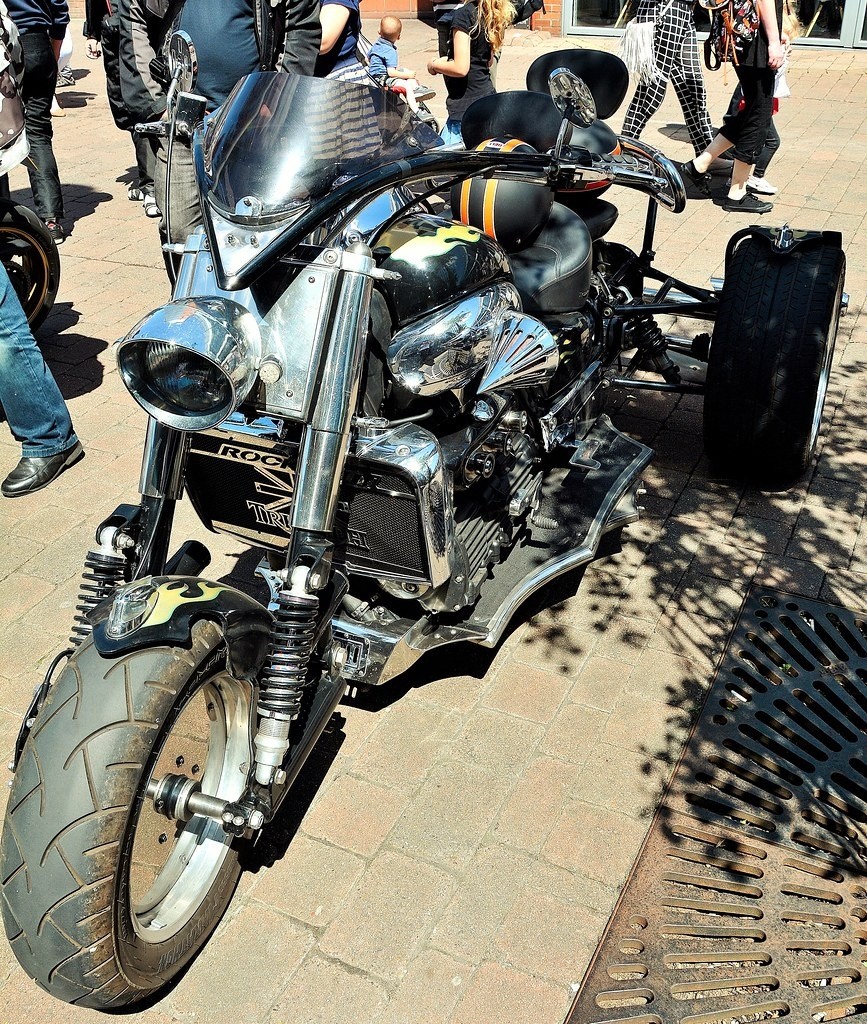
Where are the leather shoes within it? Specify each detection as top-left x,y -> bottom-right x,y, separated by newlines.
1,440 -> 83,496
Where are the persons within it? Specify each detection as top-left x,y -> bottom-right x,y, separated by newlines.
0,0 -> 803,499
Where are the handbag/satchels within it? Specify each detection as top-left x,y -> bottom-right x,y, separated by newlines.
614,14 -> 669,85
510,0 -> 543,24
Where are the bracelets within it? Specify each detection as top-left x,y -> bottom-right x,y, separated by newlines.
433,59 -> 437,74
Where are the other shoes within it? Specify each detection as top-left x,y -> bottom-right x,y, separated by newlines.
45,220 -> 65,244
708,157 -> 735,172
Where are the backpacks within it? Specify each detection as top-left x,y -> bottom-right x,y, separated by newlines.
696,0 -> 761,70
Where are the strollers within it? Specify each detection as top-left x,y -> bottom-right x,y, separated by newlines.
358,32 -> 439,149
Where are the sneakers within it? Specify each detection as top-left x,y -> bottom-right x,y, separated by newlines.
722,190 -> 773,214
726,177 -> 752,188
55,66 -> 75,87
414,85 -> 436,102
680,160 -> 712,199
746,175 -> 777,194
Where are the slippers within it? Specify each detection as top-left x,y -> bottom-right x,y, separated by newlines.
128,188 -> 144,201
143,201 -> 159,217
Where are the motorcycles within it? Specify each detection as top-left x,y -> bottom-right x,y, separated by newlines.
0,27 -> 849,1010
